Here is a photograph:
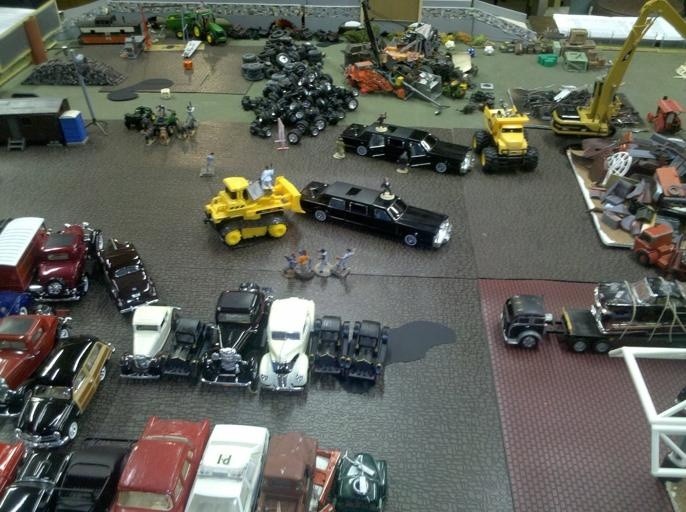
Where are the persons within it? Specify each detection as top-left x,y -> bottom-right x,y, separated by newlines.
1,3 -> 686,512
